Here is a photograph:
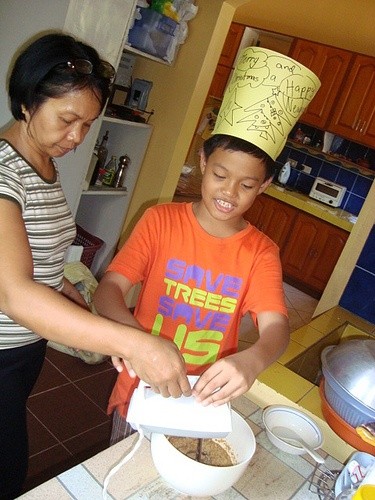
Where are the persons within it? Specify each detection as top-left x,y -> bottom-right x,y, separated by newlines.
0,32 -> 192,500
93,131 -> 290,449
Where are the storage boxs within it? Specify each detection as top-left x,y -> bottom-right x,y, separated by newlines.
71,223 -> 104,270
129,1 -> 181,59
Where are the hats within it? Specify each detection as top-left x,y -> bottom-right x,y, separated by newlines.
210,47 -> 321,163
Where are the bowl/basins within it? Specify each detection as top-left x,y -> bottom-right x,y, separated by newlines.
261,403 -> 324,455
321,339 -> 375,428
149,408 -> 257,497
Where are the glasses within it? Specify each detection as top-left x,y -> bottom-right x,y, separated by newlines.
37,59 -> 117,86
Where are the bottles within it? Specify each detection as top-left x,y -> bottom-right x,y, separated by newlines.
111,155 -> 129,189
95,168 -> 105,186
101,156 -> 117,186
90,136 -> 109,187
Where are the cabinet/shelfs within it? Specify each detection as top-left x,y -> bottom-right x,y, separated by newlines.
280,211 -> 349,291
210,24 -> 247,98
281,39 -> 353,129
328,56 -> 375,148
244,194 -> 293,250
50,0 -> 165,294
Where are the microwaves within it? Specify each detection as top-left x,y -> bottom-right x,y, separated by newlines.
308,176 -> 347,208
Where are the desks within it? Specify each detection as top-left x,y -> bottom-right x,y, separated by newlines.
13,392 -> 347,500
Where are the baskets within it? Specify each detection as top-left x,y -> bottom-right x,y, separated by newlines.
71,224 -> 103,270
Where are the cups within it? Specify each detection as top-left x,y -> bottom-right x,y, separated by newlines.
351,485 -> 375,500
335,489 -> 356,500
335,452 -> 375,497
